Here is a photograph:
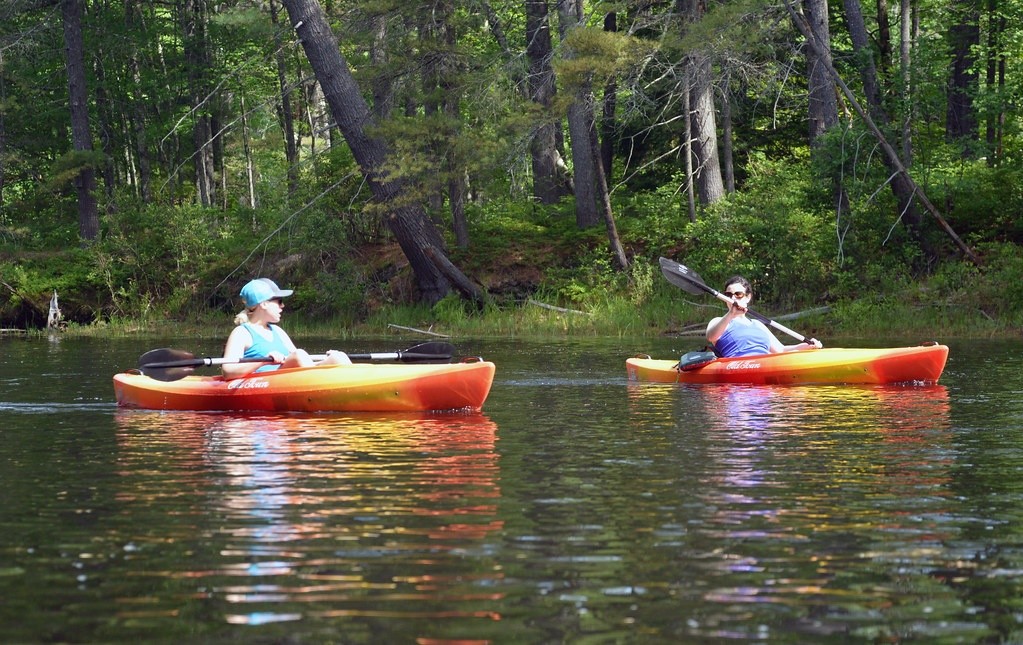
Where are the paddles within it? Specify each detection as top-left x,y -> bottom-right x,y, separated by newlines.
137,341 -> 455,382
659,256 -> 814,345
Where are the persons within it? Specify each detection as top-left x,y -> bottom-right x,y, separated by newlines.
222,278 -> 352,380
706,276 -> 823,358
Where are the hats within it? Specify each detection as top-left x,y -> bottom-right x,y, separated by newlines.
239,278 -> 294,308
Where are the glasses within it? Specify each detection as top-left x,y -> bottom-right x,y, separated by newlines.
269,298 -> 283,305
724,291 -> 747,299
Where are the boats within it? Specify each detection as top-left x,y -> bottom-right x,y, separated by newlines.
115,358 -> 495,413
624,343 -> 949,387
